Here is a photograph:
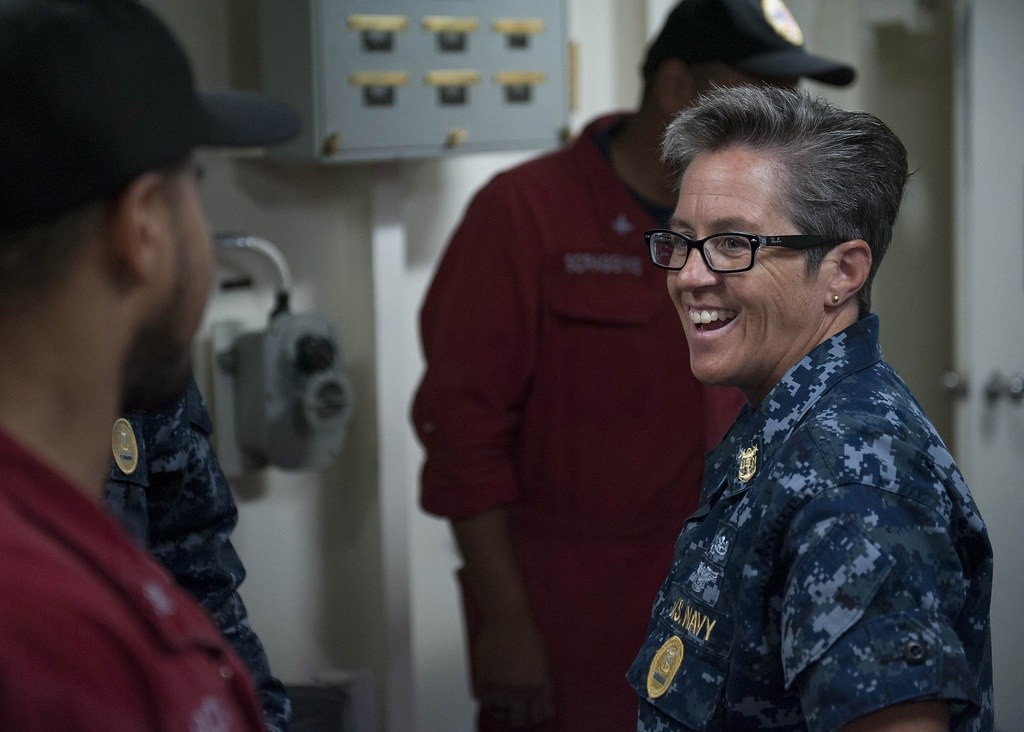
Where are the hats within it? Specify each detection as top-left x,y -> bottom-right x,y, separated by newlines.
639,0 -> 860,88
1,1 -> 304,210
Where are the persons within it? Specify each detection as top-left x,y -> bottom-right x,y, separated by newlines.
0,0 -> 304,731
100,339 -> 296,732
409,0 -> 858,732
626,88 -> 994,732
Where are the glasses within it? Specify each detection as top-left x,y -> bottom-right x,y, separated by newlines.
644,227 -> 828,273
